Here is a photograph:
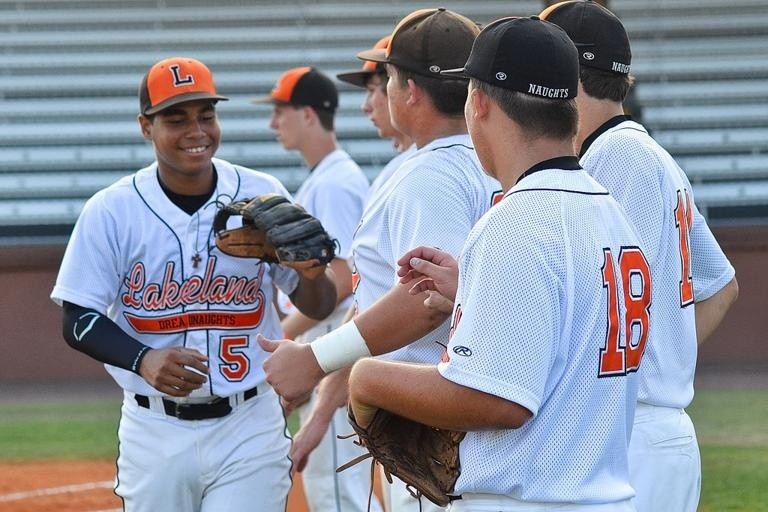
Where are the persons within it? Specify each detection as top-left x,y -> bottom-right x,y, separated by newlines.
345,16 -> 652,512
254,1 -> 503,512
50,58 -> 338,511
533,2 -> 742,512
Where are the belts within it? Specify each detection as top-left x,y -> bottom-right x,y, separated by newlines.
135,387 -> 257,420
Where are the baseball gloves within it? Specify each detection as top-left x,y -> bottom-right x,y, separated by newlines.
213,194 -> 336,270
347,400 -> 466,508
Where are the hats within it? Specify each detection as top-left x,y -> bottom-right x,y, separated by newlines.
439,15 -> 578,98
139,58 -> 229,116
336,35 -> 391,87
252,67 -> 337,110
359,8 -> 480,78
539,0 -> 631,74
